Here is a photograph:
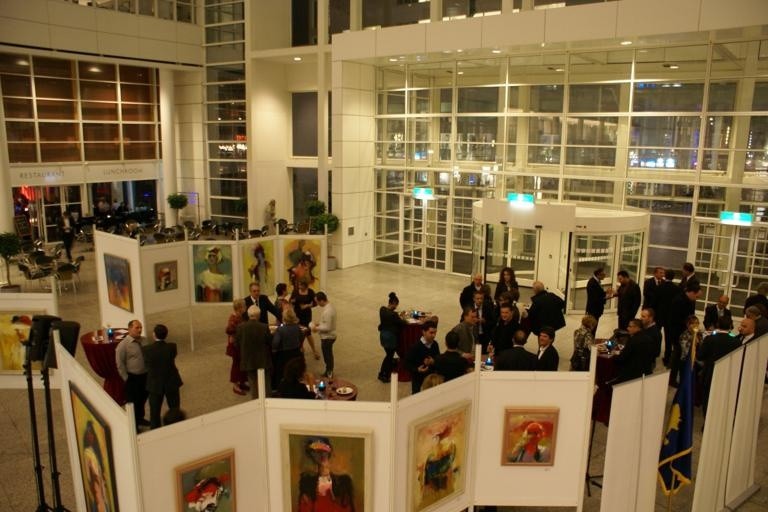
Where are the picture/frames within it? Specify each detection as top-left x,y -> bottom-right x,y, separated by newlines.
103,252 -> 133,313
172,447 -> 238,511
68,380 -> 119,511
499,406 -> 561,467
278,422 -> 376,512
407,398 -> 473,512
0,307 -> 57,377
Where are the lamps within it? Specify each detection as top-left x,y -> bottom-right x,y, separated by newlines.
40,320 -> 82,512
20,315 -> 63,512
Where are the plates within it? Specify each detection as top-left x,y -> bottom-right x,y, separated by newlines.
336,386 -> 354,395
417,311 -> 429,317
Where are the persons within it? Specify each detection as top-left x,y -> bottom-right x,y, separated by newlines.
14,194 -> 129,217
223,280 -> 339,397
58,209 -> 83,263
375,268 -> 567,393
114,320 -> 184,433
572,266 -> 767,437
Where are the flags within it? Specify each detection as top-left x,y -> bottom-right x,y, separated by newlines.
656,325 -> 699,497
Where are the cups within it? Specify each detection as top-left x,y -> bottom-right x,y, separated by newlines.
319,384 -> 326,397
107,327 -> 113,343
606,341 -> 613,350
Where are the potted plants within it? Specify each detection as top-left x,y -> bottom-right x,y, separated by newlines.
313,213 -> 339,271
0,232 -> 23,294
167,193 -> 188,233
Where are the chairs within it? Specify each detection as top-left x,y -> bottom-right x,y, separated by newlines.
20,210 -> 301,296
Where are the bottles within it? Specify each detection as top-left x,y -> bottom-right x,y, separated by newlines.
97,329 -> 103,342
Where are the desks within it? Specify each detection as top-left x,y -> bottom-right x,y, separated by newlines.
81,328 -> 130,406
589,338 -> 625,421
299,378 -> 359,400
397,310 -> 439,381
